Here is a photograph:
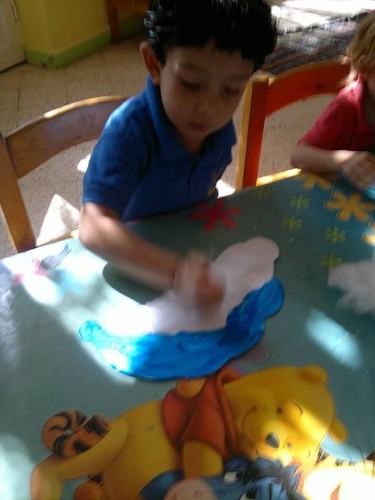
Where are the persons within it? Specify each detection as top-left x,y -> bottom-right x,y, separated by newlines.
289,9 -> 375,191
78,0 -> 279,306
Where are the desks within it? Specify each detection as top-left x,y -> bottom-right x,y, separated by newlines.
0,171 -> 375,500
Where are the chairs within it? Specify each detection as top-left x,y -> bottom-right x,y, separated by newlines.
0,95 -> 131,254
234,60 -> 350,193
